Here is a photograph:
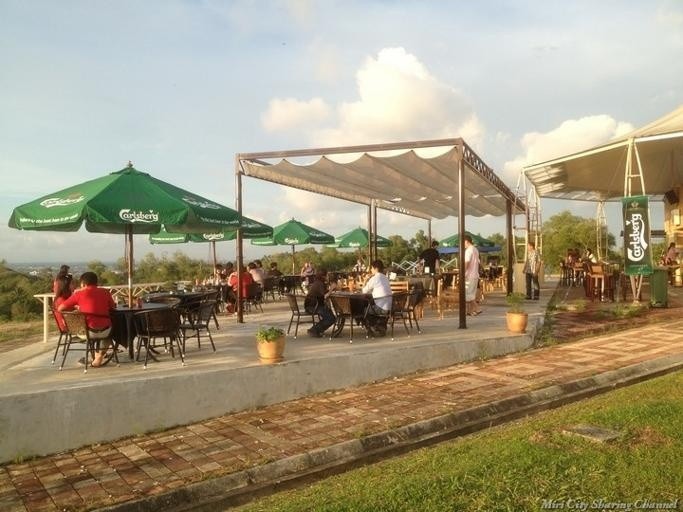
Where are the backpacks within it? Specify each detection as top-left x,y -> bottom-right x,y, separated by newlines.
224,283 -> 237,304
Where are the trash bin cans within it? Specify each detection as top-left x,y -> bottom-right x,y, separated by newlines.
649,269 -> 670,309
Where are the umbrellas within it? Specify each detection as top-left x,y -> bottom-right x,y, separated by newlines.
149,215 -> 273,276
325,226 -> 393,260
439,231 -> 495,248
8,160 -> 273,309
251,217 -> 335,276
438,244 -> 502,254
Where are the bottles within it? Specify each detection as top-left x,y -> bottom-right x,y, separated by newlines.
135,297 -> 143,308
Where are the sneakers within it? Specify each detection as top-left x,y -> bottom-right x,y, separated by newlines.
306,326 -> 323,338
524,295 -> 539,301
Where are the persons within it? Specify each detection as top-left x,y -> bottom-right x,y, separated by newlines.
464,235 -> 482,317
53,265 -> 117,367
195,259 -> 286,315
561,247 -> 598,276
301,259 -> 393,338
665,242 -> 679,286
417,241 -> 440,300
523,241 -> 542,301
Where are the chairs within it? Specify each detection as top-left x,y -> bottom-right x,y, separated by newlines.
284,260 -> 506,342
557,256 -> 643,303
52,270 -> 282,368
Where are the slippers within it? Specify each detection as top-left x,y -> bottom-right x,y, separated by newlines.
465,310 -> 482,316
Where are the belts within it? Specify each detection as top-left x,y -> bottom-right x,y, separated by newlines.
85,326 -> 109,334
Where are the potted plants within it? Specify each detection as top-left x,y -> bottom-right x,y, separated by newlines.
254,326 -> 287,359
506,292 -> 528,333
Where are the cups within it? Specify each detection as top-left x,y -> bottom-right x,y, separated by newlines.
348,281 -> 354,291
111,295 -> 118,308
